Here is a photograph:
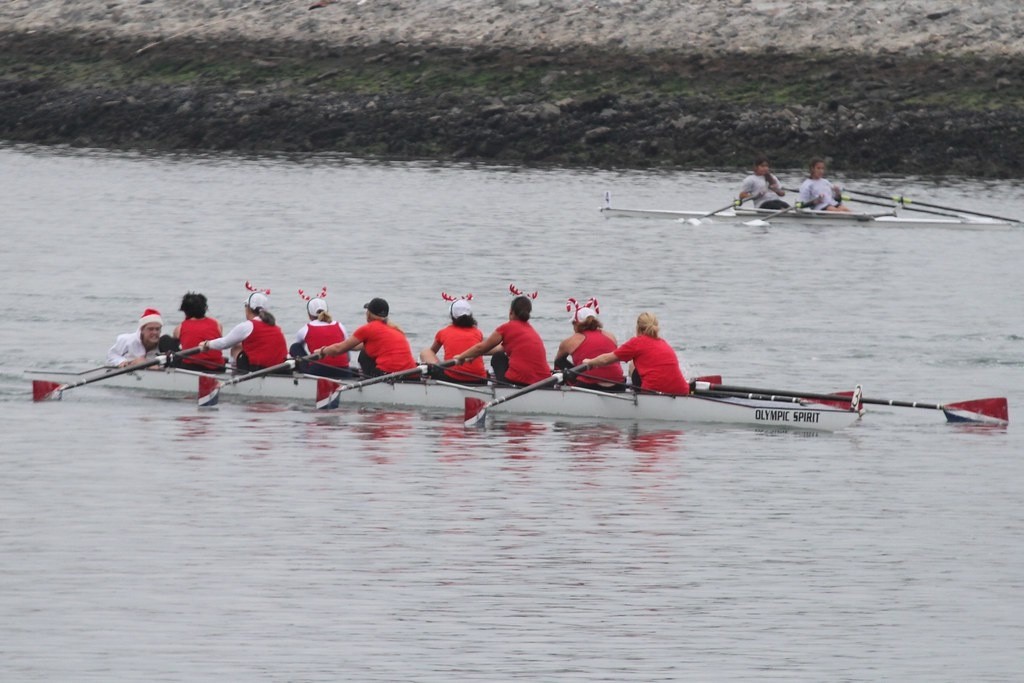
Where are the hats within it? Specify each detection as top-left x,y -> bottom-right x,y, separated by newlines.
306,298 -> 327,317
363,298 -> 388,318
569,307 -> 597,322
138,309 -> 163,328
450,300 -> 471,319
244,291 -> 268,310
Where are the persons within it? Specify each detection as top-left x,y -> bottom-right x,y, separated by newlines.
420,293 -> 487,384
454,284 -> 551,386
107,308 -> 170,370
199,282 -> 288,374
289,287 -> 351,378
798,158 -> 849,211
582,313 -> 690,394
174,293 -> 226,373
739,156 -> 790,210
554,297 -> 627,392
314,298 -> 419,381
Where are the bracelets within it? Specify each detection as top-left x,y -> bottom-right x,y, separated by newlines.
204,340 -> 208,349
321,347 -> 326,356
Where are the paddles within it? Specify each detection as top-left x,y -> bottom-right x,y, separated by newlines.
690,381 -> 1010,424
462,363 -> 595,430
314,360 -> 467,410
701,192 -> 763,219
687,374 -> 720,386
760,185 -> 970,221
687,389 -> 860,415
32,348 -> 201,405
836,188 -> 1023,226
197,353 -> 325,407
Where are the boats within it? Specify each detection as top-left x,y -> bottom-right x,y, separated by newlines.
600,189 -> 901,221
99,357 -> 875,435
